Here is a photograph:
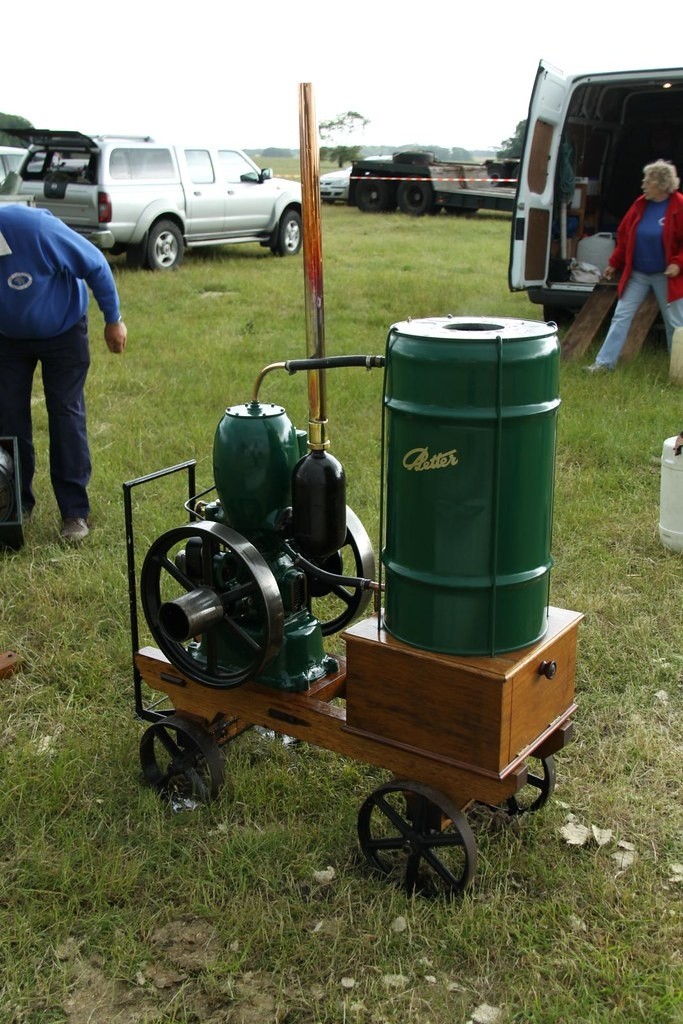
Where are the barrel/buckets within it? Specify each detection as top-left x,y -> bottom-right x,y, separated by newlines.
577,231 -> 615,274
659,436 -> 683,551
383,316 -> 561,656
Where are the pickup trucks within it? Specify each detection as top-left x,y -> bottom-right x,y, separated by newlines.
0,128 -> 302,273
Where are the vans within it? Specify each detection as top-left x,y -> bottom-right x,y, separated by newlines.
507,59 -> 683,325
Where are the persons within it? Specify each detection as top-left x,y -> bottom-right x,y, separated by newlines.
581,158 -> 683,373
0,203 -> 128,547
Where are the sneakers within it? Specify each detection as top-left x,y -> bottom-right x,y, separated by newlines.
60,515 -> 88,541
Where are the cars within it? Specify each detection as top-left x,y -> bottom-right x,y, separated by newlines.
319,155 -> 393,205
0,146 -> 59,187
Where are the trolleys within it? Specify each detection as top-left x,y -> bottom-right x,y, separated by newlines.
120,82 -> 587,898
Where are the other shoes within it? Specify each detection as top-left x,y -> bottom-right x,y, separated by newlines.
587,363 -> 608,372
